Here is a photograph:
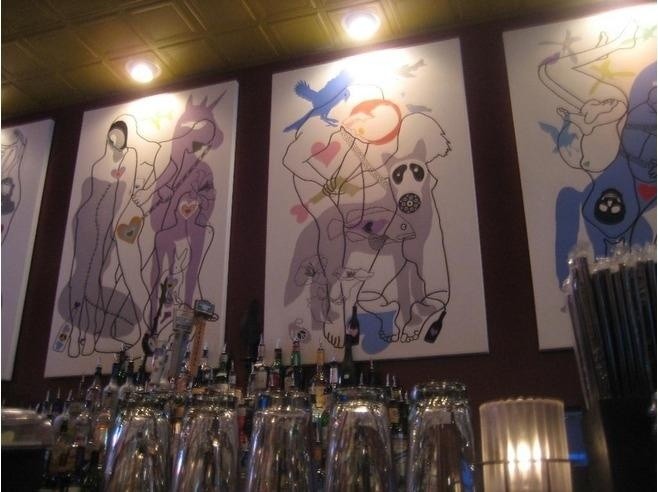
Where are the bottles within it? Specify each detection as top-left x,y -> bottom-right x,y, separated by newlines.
40,448 -> 99,490
423,310 -> 447,343
28,331 -> 408,476
345,305 -> 359,345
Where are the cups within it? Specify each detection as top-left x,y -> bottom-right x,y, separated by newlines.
405,383 -> 479,492
170,394 -> 237,491
101,398 -> 169,490
477,396 -> 571,491
581,397 -> 656,491
323,385 -> 394,492
245,391 -> 314,491
